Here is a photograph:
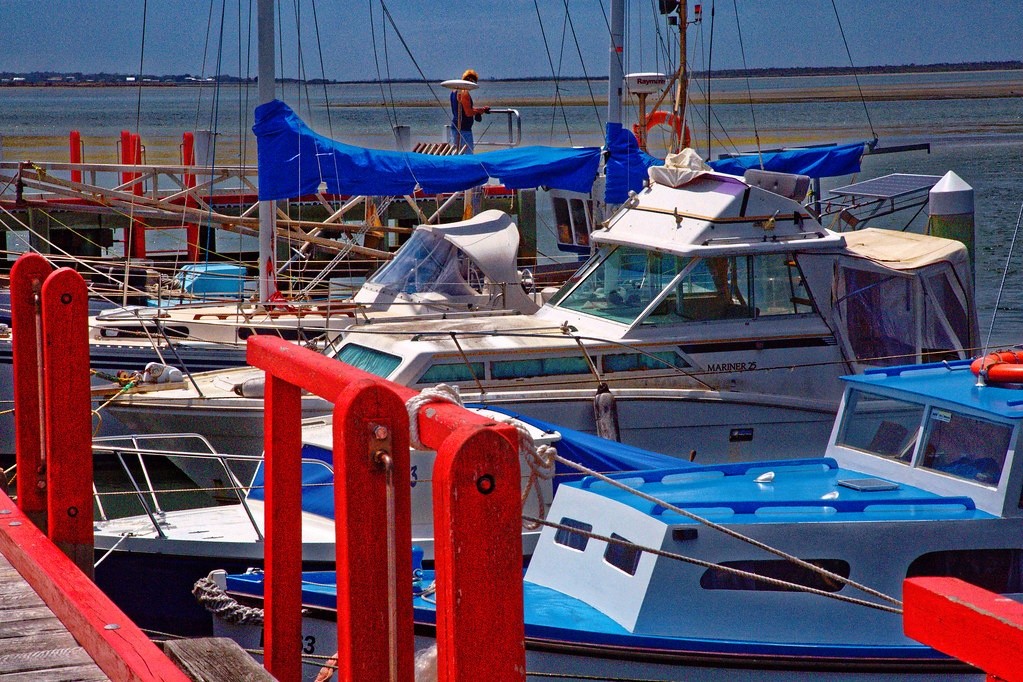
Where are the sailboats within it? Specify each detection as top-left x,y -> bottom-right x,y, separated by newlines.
0,0 -> 1023,682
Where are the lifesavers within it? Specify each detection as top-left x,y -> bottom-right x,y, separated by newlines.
635,111 -> 692,159
971,348 -> 1023,383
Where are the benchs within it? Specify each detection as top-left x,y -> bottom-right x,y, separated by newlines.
744,169 -> 811,203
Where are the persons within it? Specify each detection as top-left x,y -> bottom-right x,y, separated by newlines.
450,70 -> 490,154
